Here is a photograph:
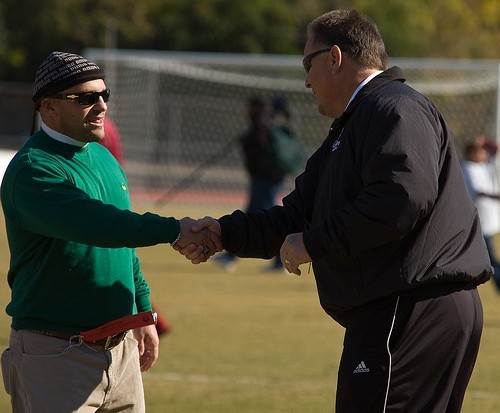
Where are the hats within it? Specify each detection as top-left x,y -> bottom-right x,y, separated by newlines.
31,50 -> 105,99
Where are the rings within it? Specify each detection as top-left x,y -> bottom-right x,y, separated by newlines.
284,258 -> 289,264
202,245 -> 212,256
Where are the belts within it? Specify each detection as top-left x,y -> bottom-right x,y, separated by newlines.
29,328 -> 130,350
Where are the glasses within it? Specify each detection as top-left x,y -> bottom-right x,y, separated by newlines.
302,47 -> 347,73
45,89 -> 111,105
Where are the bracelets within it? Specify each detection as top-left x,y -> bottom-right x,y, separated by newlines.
170,220 -> 183,245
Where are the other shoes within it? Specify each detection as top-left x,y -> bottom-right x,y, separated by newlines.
211,254 -> 236,273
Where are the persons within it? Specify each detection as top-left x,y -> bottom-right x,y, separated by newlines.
97,113 -> 171,336
211,90 -> 299,272
461,135 -> 499,294
174,8 -> 500,412
1,50 -> 224,413
